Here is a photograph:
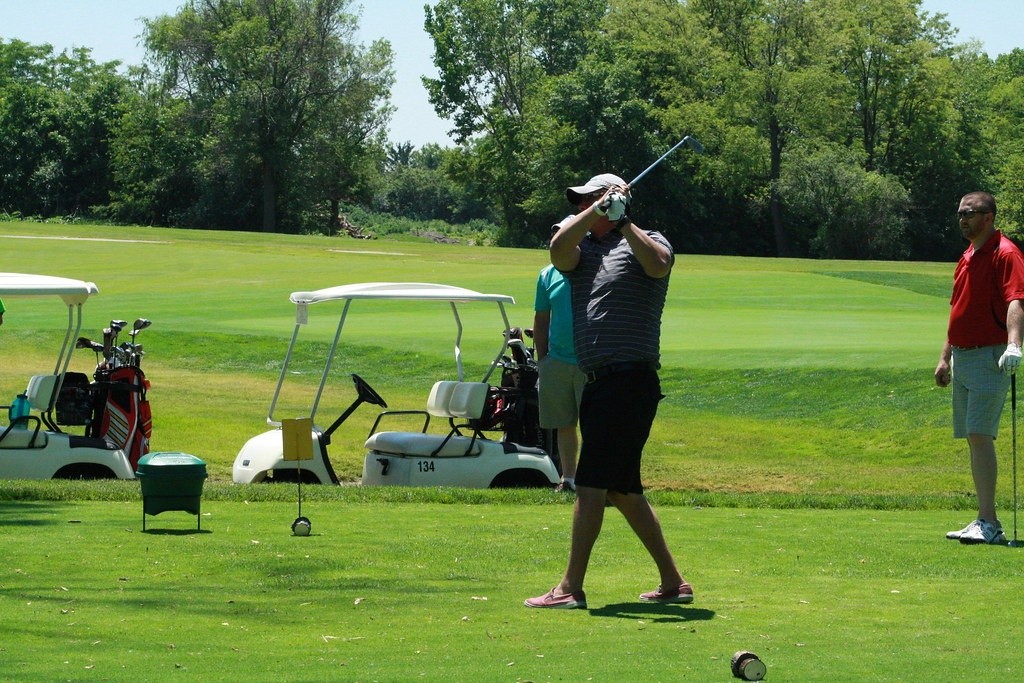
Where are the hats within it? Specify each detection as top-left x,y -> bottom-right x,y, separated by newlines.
567,174 -> 632,206
551,215 -> 591,236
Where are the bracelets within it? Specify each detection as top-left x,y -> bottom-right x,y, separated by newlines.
616,215 -> 631,231
593,201 -> 608,216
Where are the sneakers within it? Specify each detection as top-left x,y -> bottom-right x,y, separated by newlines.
947,519 -> 1006,541
960,520 -> 999,544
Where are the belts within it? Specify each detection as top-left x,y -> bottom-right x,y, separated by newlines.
586,361 -> 657,384
957,341 -> 1008,351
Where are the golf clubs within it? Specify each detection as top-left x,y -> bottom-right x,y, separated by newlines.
1008,370 -> 1019,548
490,325 -> 539,387
74,318 -> 152,376
599,135 -> 704,214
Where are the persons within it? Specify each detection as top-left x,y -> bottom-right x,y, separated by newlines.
533,215 -> 588,492
523,173 -> 694,609
934,192 -> 1024,543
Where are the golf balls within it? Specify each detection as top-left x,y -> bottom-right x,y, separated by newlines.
939,372 -> 950,386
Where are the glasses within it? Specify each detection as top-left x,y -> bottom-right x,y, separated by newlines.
956,208 -> 998,218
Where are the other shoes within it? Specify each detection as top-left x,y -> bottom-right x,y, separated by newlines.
554,481 -> 576,493
523,587 -> 587,609
639,583 -> 694,604
